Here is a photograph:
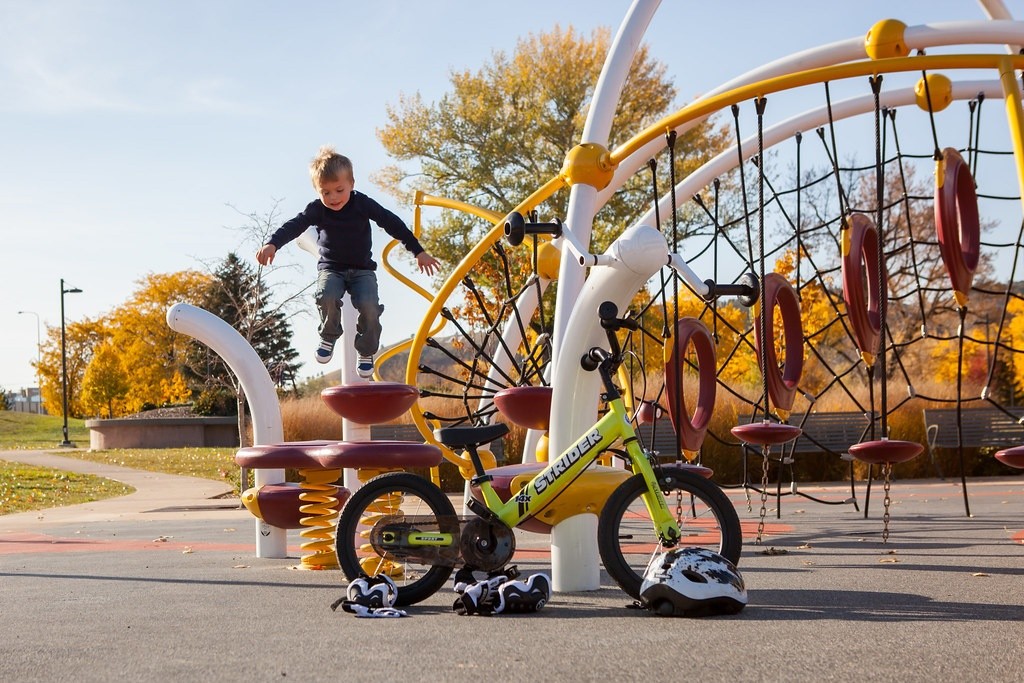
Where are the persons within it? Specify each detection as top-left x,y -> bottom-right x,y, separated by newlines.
255,146 -> 442,379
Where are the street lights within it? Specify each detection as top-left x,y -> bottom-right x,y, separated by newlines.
59,279 -> 84,448
18,310 -> 42,415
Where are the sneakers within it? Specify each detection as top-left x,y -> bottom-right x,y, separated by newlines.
313,340 -> 336,364
355,349 -> 375,378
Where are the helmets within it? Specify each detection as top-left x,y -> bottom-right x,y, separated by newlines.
639,544 -> 748,616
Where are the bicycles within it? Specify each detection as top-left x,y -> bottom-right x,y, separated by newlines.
333,299 -> 744,610
